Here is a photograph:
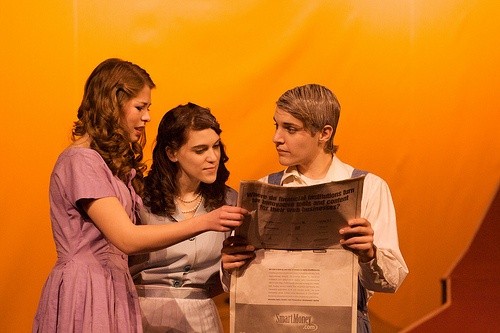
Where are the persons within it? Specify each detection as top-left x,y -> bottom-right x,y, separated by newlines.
127,102 -> 240,333
220,84 -> 410,333
31,58 -> 249,333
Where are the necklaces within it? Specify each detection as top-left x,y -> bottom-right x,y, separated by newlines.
174,190 -> 201,214
